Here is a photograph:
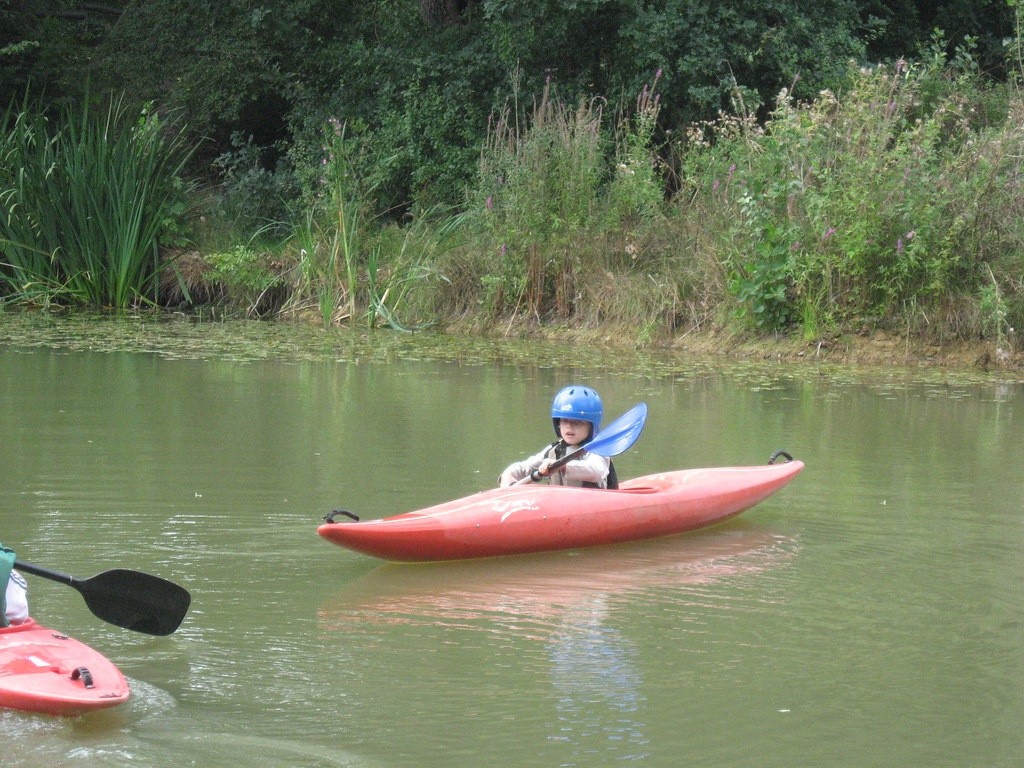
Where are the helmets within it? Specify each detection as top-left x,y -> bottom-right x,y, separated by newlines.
551,384 -> 603,440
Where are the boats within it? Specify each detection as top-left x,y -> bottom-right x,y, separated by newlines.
0,613 -> 130,718
316,449 -> 807,563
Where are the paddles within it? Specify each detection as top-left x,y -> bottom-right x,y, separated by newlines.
514,403 -> 647,484
13,560 -> 191,637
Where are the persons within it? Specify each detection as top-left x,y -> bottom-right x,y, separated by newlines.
498,385 -> 617,489
0,543 -> 27,626
